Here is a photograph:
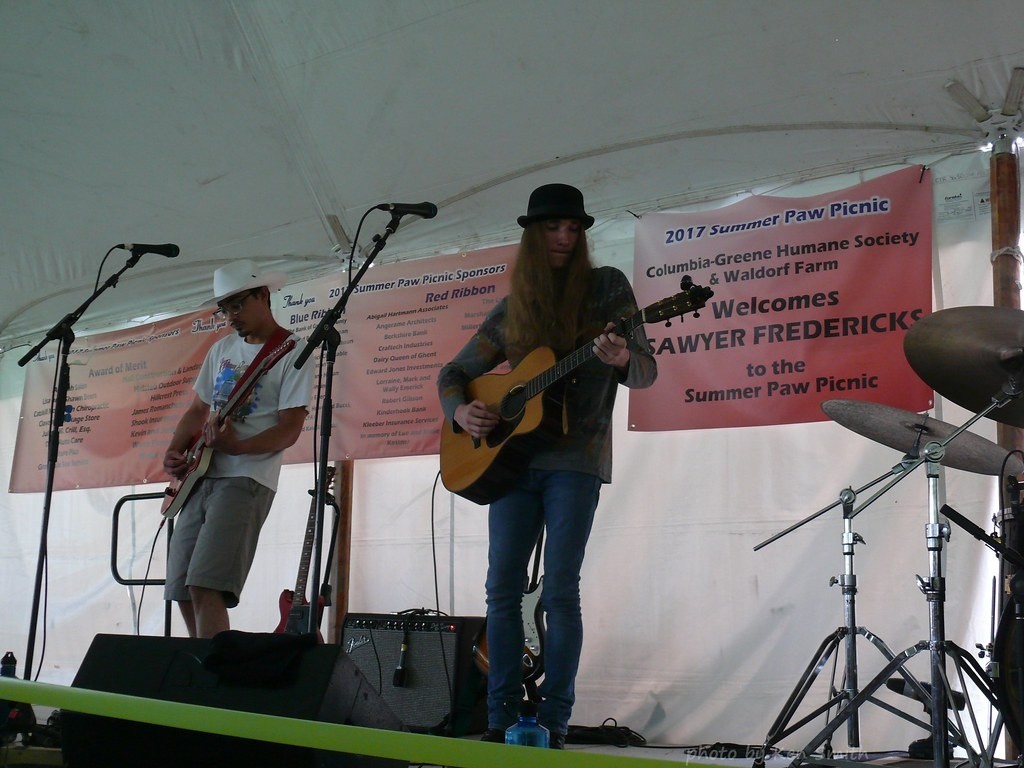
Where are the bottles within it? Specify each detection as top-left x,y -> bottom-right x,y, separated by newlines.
504,703 -> 550,748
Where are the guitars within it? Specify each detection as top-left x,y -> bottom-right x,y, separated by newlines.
439,285 -> 712,505
273,466 -> 337,645
160,338 -> 296,520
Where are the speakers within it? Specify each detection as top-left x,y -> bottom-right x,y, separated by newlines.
59,633 -> 413,768
340,612 -> 490,739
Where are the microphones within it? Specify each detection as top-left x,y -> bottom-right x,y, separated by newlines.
115,243 -> 180,258
378,202 -> 438,219
393,634 -> 409,688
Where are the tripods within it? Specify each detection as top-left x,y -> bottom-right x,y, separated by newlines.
750,368 -> 1016,768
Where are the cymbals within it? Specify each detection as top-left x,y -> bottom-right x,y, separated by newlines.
904,305 -> 1024,430
820,399 -> 1024,477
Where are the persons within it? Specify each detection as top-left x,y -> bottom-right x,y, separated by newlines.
163,260 -> 320,638
438,184 -> 657,749
